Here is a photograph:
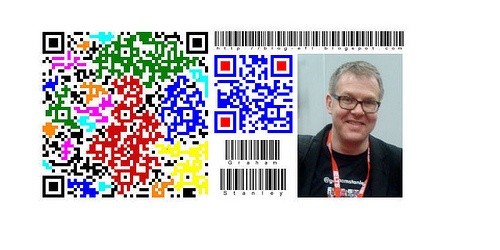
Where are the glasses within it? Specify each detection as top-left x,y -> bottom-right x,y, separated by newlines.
329,93 -> 380,114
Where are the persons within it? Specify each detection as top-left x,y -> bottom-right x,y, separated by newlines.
297,60 -> 403,198
